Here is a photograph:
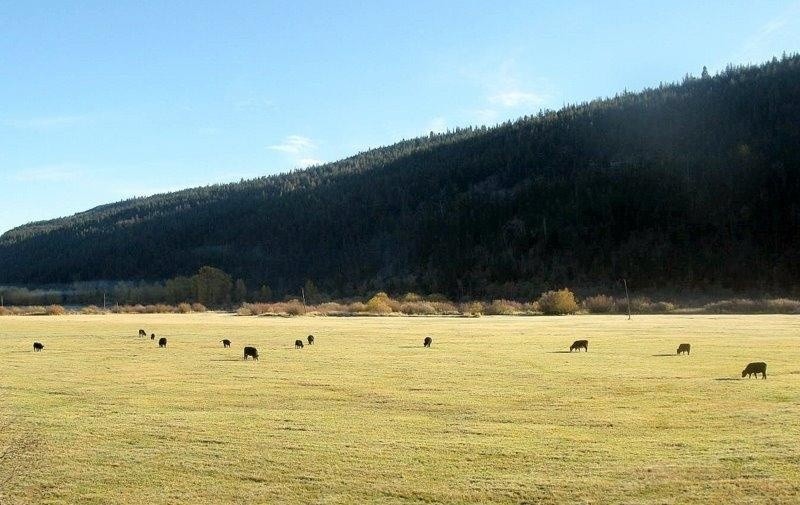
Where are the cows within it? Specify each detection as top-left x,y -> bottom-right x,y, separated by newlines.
741,361 -> 766,380
220,339 -> 233,348
425,337 -> 432,348
308,335 -> 315,346
33,342 -> 45,352
139,329 -> 147,337
151,333 -> 155,340
569,340 -> 589,352
244,347 -> 260,361
677,343 -> 691,356
295,340 -> 304,348
159,337 -> 167,347
